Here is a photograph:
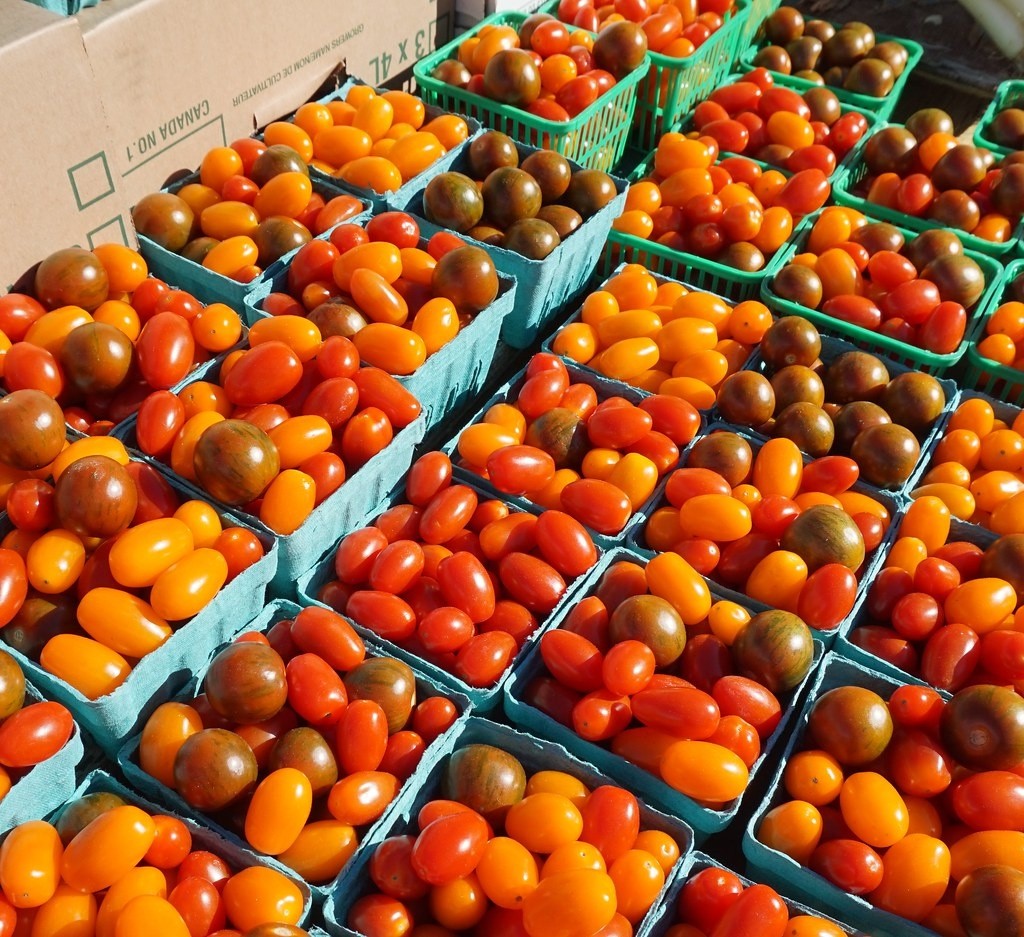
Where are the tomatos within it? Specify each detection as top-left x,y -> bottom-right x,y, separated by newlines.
0,0 -> 1024,937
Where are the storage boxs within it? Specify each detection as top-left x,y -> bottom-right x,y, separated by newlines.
2,1 -> 1024,937
